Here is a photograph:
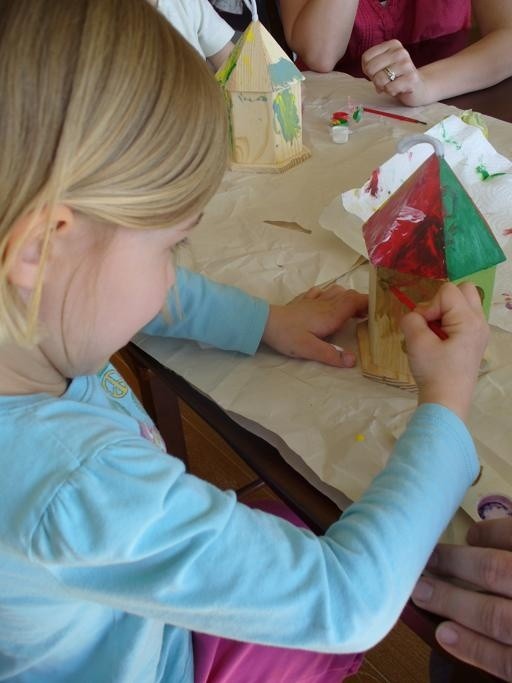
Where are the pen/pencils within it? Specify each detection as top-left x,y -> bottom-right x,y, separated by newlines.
363,108 -> 428,125
379,277 -> 451,341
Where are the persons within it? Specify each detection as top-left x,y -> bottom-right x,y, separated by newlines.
140,0 -> 239,79
4,0 -> 493,680
403,515 -> 512,682
274,0 -> 511,107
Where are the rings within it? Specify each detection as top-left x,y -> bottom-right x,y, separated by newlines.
383,67 -> 397,80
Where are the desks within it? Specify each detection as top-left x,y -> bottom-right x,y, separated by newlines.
116,24 -> 512,683
